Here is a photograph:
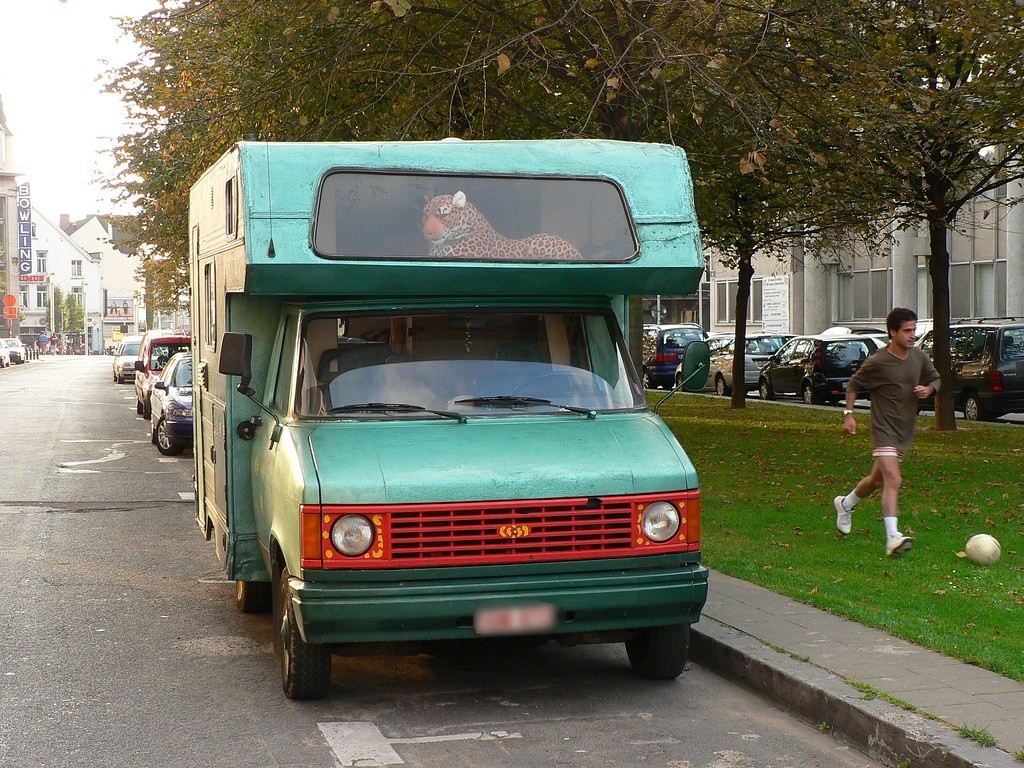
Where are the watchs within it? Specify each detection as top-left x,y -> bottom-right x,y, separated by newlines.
844,410 -> 853,415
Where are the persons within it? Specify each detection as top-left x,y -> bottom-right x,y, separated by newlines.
834,308 -> 941,559
40,331 -> 59,355
110,300 -> 128,314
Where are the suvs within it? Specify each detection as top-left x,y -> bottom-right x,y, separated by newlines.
912,316 -> 1024,423
643,321 -> 709,390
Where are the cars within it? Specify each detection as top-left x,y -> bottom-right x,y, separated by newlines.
0,339 -> 13,368
3,338 -> 27,365
819,325 -> 893,354
675,332 -> 790,397
758,335 -> 882,405
150,351 -> 194,457
108,334 -> 144,384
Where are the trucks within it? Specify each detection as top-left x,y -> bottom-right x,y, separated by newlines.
187,137 -> 711,701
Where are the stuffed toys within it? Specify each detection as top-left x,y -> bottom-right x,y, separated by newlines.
422,191 -> 583,259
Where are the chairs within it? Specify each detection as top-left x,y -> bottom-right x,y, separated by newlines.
317,349 -> 373,410
493,340 -> 544,397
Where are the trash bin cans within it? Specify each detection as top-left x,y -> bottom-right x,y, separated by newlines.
16,353 -> 21,364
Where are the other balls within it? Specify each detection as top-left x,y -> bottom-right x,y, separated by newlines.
966,532 -> 1001,566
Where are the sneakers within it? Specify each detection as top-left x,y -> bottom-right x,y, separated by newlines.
834,495 -> 855,536
884,532 -> 915,559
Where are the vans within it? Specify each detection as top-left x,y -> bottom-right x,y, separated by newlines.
134,330 -> 192,422
